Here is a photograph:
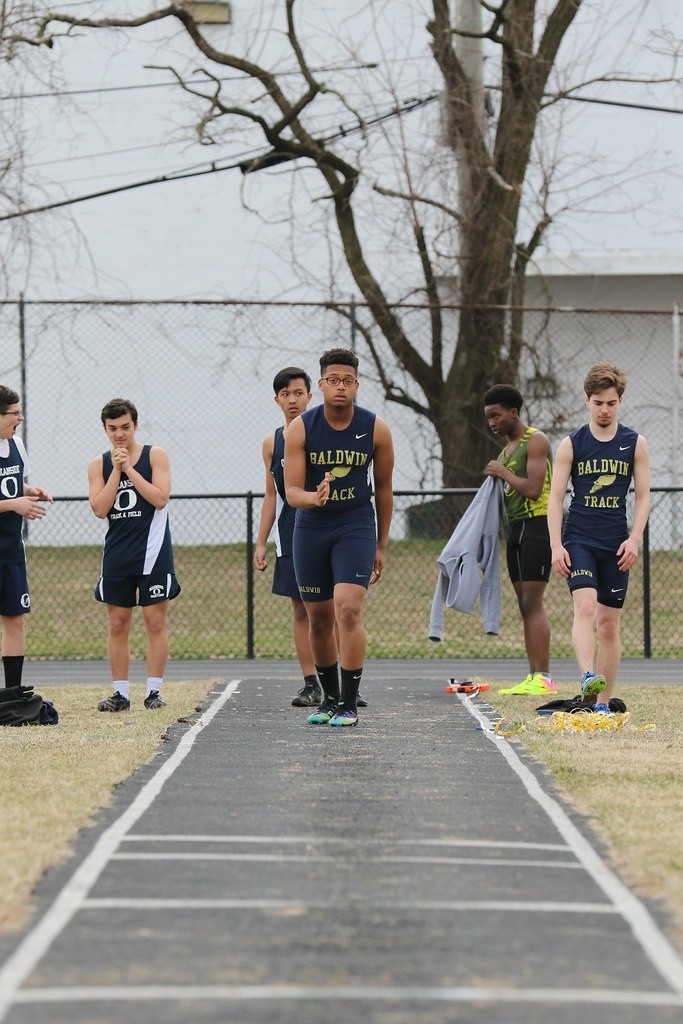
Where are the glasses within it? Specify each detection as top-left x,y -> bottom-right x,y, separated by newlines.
322,377 -> 357,387
4,408 -> 23,416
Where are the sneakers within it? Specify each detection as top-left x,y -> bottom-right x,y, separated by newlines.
581,670 -> 607,704
498,674 -> 532,695
98,691 -> 131,711
144,690 -> 167,709
329,702 -> 359,726
513,674 -> 557,695
292,682 -> 322,706
592,703 -> 610,717
306,696 -> 338,724
356,689 -> 367,706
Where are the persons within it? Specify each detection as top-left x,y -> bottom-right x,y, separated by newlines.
480,384 -> 559,695
87,400 -> 181,713
283,348 -> 394,726
0,384 -> 54,695
254,367 -> 372,706
546,363 -> 650,714
